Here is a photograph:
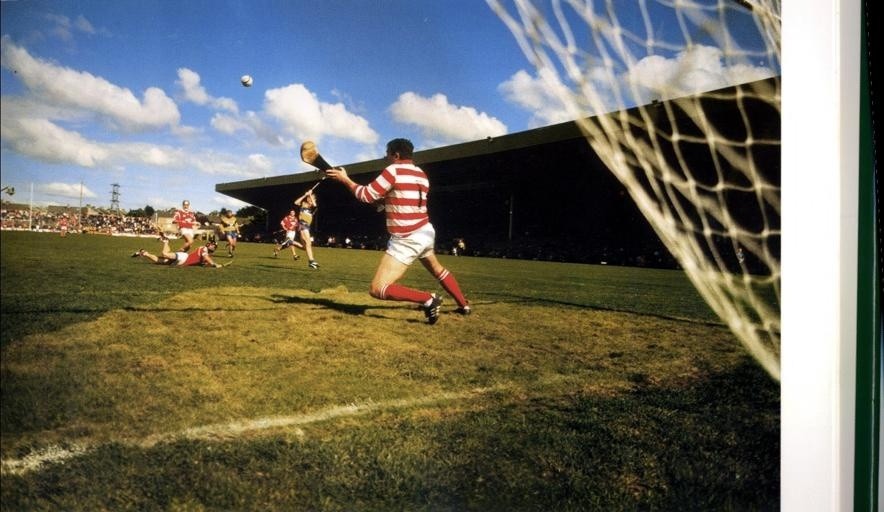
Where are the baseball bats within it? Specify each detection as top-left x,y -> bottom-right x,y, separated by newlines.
216,261 -> 232,268
311,173 -> 328,191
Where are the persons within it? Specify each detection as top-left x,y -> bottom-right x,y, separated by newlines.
131,199 -> 241,268
324,139 -> 471,325
345,236 -> 352,248
326,234 -> 337,248
451,240 -> 466,256
0,205 -> 162,238
273,190 -> 323,269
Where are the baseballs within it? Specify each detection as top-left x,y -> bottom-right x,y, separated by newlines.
241,75 -> 254,87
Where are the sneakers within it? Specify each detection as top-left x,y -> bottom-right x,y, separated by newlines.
422,292 -> 442,325
280,237 -> 291,249
456,305 -> 472,315
227,250 -> 320,270
129,231 -> 170,257
